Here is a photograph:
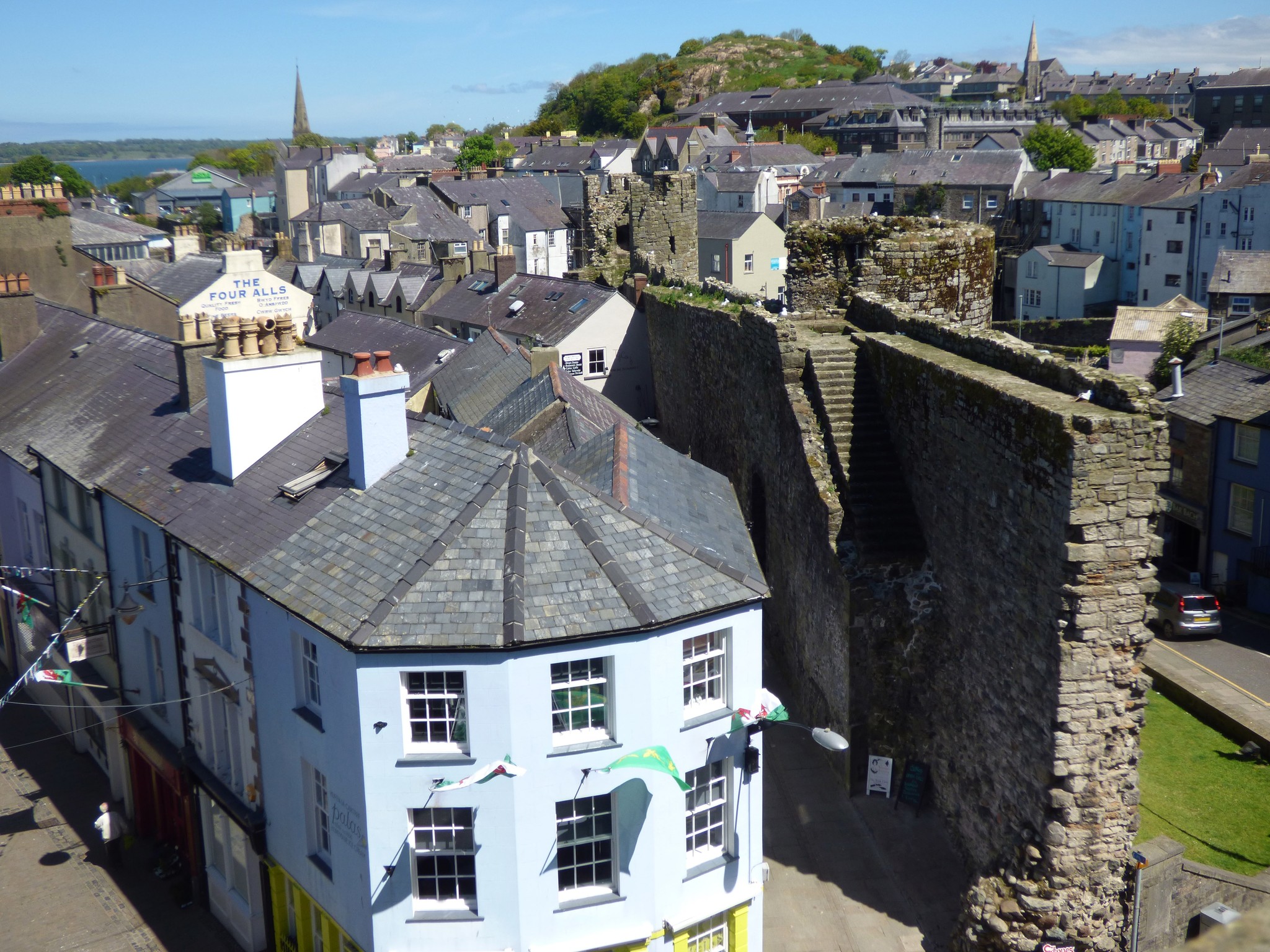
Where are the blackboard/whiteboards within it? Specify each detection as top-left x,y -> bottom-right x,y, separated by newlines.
898,759 -> 931,808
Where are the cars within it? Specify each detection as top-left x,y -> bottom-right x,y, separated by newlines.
1143,582 -> 1223,641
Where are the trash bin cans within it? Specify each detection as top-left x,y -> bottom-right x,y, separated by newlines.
1199,901 -> 1241,936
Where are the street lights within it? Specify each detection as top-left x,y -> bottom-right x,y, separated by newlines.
759,281 -> 767,300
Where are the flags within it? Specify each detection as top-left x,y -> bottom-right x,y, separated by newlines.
600,745 -> 692,792
430,754 -> 526,793
33,669 -> 82,687
453,698 -> 467,741
16,592 -> 39,630
553,691 -> 604,723
728,688 -> 789,734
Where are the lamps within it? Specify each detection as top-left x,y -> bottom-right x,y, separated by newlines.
745,718 -> 849,773
638,417 -> 660,426
1180,312 -> 1221,321
109,576 -> 182,626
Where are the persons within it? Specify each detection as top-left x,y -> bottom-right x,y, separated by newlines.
94,802 -> 129,866
